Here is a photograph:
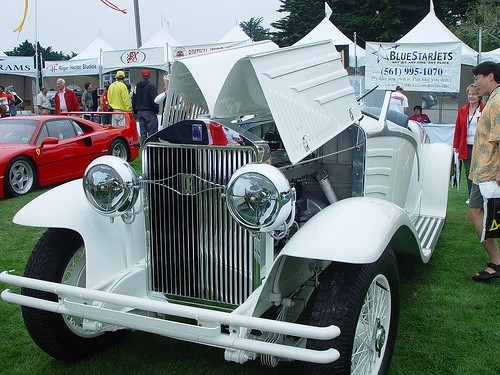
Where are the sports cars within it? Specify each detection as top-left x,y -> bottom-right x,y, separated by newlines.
0,111 -> 139,200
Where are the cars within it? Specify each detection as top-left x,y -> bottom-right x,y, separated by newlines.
0,40 -> 460,375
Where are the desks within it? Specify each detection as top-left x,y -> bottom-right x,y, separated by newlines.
422,123 -> 455,144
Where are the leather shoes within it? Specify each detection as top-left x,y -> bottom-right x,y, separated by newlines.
472,262 -> 500,282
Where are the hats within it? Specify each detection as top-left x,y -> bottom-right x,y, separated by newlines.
142,69 -> 151,79
116,71 -> 125,78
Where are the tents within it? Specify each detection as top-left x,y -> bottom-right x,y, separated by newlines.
291,13 -> 500,122
0,23 -> 265,117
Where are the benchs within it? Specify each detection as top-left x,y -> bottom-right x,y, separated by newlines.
240,112 -> 366,215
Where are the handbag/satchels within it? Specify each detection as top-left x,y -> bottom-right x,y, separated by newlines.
9,92 -> 23,107
479,180 -> 500,243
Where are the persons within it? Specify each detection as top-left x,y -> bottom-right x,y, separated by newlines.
0,70 -> 171,150
388,60 -> 500,281
